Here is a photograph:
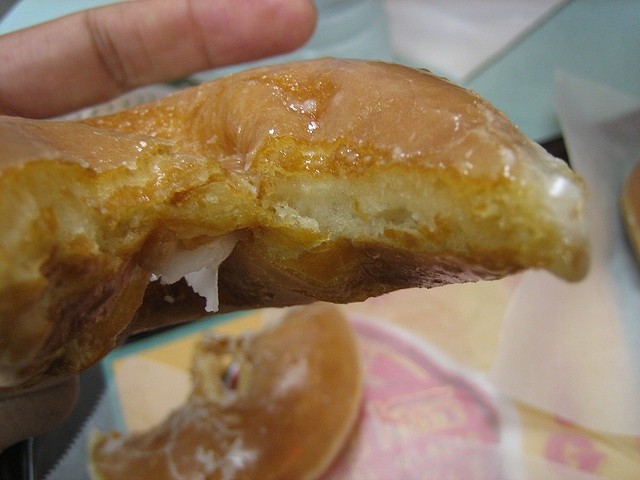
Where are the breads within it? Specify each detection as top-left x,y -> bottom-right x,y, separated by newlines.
90,300 -> 364,480
0,57 -> 590,399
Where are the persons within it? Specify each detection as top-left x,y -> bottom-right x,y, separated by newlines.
0,1 -> 318,451
